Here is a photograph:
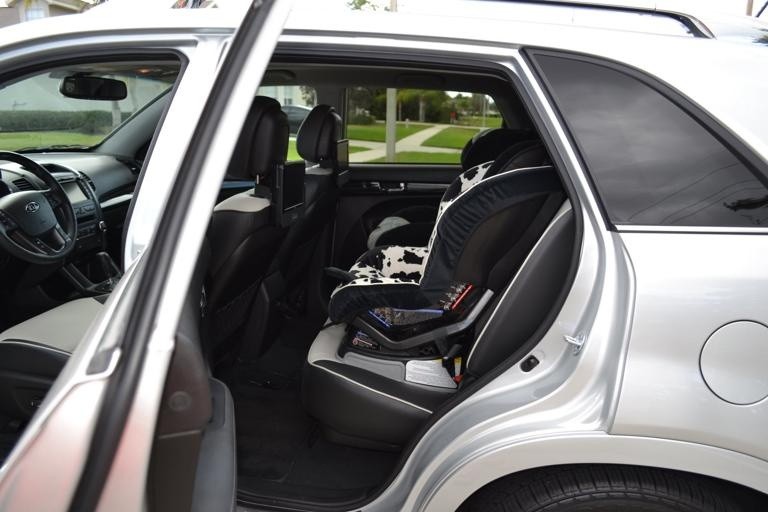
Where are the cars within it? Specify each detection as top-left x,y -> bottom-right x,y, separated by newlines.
277,104 -> 311,136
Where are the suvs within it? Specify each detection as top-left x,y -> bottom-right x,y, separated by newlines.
0,1 -> 768,512
